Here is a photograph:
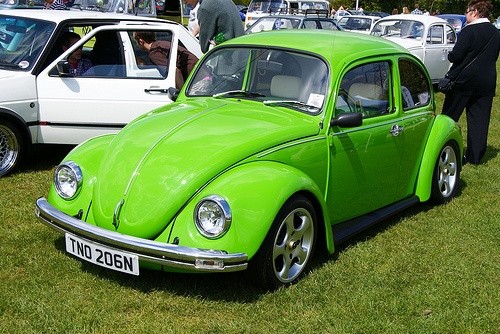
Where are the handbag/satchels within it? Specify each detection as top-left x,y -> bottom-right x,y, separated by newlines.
438,77 -> 455,93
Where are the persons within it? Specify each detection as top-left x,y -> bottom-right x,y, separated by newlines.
43,0 -> 68,10
188,2 -> 201,39
134,23 -> 213,95
440,0 -> 500,165
57,32 -> 95,76
392,4 -> 430,16
183,0 -> 250,92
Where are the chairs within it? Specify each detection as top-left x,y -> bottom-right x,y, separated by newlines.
347,84 -> 388,117
269,75 -> 304,100
90,40 -> 117,66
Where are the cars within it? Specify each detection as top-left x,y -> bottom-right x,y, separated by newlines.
34,28 -> 462,288
243,0 -> 469,90
0,0 -> 170,21
0,9 -> 215,176
